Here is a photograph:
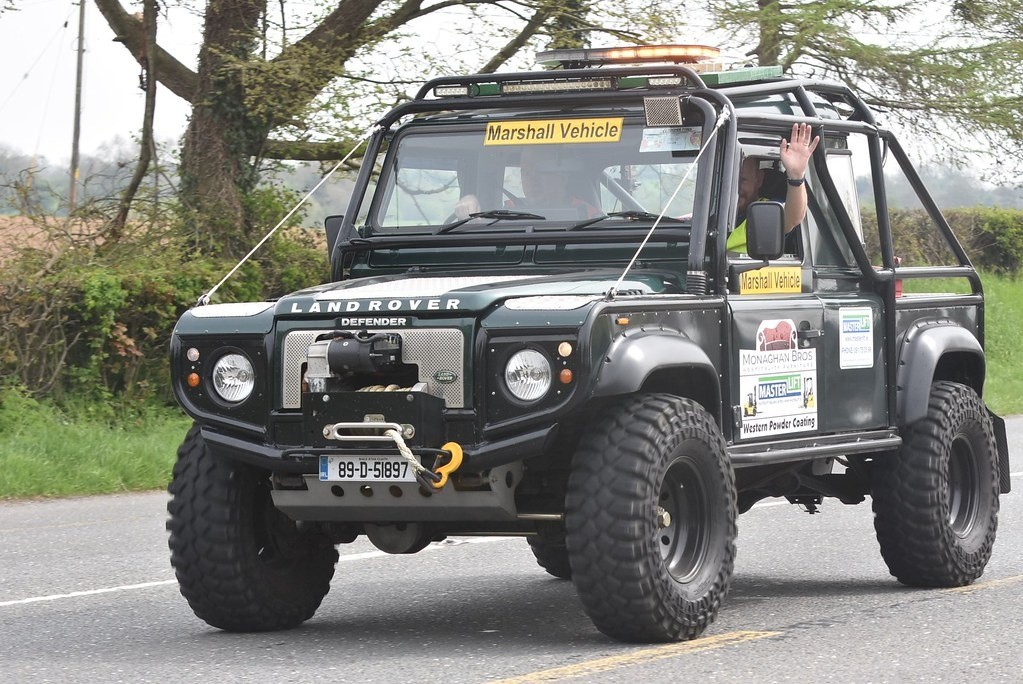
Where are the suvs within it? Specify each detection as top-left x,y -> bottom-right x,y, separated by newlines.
166,43 -> 1013,647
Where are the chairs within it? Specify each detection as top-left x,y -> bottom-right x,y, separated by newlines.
521,145 -> 601,209
757,168 -> 788,202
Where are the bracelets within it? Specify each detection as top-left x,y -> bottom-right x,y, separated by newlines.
787,175 -> 805,187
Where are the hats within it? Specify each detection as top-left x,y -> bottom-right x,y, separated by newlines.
521,145 -> 577,173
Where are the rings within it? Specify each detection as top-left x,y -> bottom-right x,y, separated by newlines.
803,143 -> 809,146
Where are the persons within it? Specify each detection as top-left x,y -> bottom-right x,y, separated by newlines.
675,122 -> 820,254
454,148 -> 604,224
